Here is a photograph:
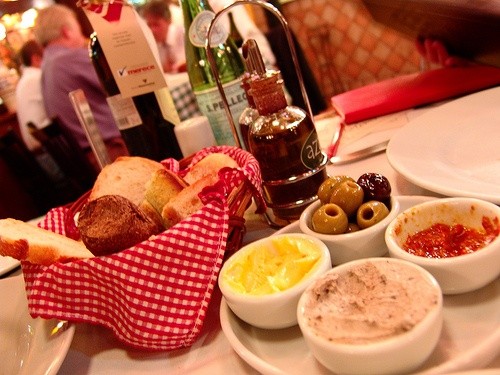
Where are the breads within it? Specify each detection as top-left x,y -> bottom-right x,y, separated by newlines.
77,153 -> 240,255
0,217 -> 95,265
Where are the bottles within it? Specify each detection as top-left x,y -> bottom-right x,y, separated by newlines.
181,0 -> 250,148
89,32 -> 184,162
237,39 -> 329,229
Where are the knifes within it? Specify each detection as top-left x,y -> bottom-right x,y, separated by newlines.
331,139 -> 389,164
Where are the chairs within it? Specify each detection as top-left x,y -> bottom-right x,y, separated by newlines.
0,117 -> 96,219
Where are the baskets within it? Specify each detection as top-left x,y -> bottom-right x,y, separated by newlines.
19,145 -> 259,291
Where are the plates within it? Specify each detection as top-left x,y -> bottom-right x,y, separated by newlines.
220,196 -> 500,375
385,86 -> 499,206
0,253 -> 75,375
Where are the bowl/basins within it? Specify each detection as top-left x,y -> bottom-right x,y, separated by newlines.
384,197 -> 495,295
296,257 -> 444,375
218,233 -> 333,329
298,198 -> 400,266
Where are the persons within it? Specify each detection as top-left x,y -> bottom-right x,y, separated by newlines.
15,40 -> 60,182
139,0 -> 187,73
34,4 -> 130,174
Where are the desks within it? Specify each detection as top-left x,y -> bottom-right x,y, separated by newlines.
62,107 -> 435,375
